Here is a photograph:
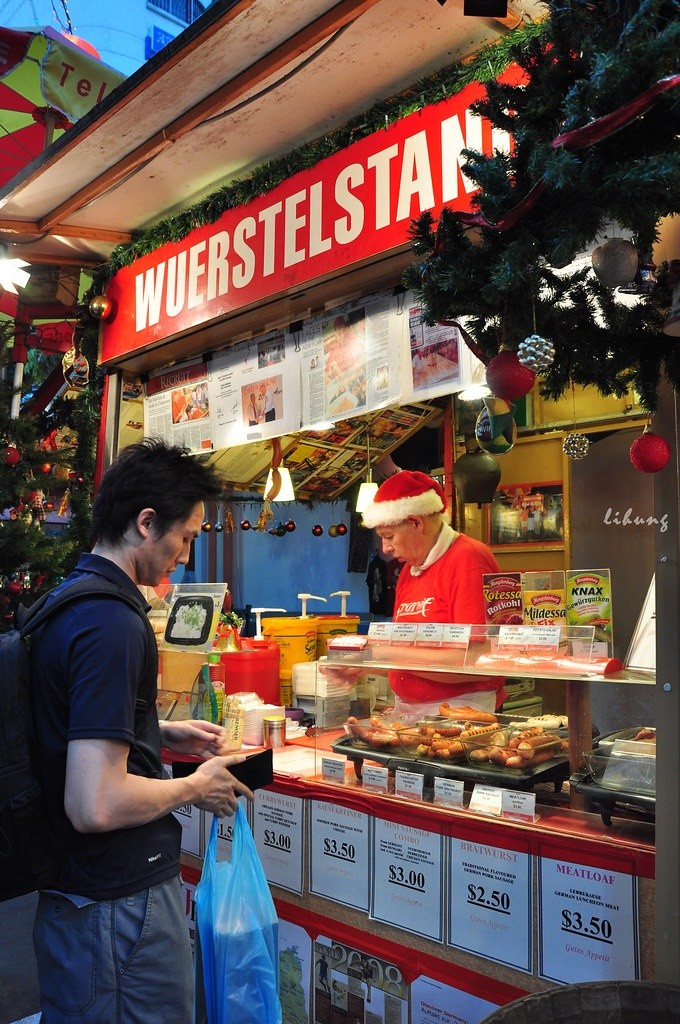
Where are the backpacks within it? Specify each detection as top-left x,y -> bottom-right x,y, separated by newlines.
0,580 -> 157,902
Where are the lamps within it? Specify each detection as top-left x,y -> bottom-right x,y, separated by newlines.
356,435 -> 378,512
263,459 -> 296,502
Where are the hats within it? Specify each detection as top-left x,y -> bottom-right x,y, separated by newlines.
362,471 -> 449,529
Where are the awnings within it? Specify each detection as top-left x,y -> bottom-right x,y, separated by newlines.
0,0 -> 552,268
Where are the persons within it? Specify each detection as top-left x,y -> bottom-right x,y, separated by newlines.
26,441 -> 256,1024
172,382 -> 209,424
410,334 -> 458,382
325,315 -> 387,406
260,385 -> 276,423
361,471 -> 508,715
248,393 -> 261,426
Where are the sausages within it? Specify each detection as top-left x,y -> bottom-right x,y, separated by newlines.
345,702 -> 572,769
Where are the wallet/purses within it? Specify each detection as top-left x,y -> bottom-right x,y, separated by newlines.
172,748 -> 274,797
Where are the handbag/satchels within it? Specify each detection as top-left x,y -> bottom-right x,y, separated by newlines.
191,799 -> 284,1024
